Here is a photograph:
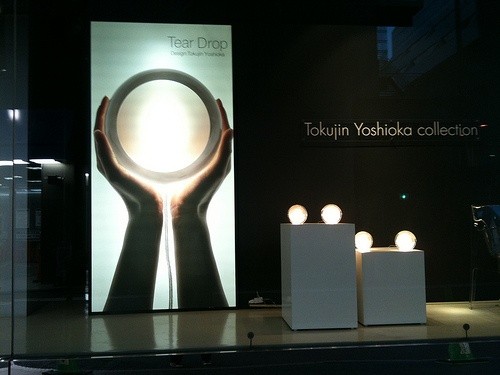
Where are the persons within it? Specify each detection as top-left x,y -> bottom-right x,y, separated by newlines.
93,94 -> 234,315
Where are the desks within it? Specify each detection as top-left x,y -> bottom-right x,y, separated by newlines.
355,249 -> 425,325
281,223 -> 358,330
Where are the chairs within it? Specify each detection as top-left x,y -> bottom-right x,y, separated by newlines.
471,205 -> 500,309
